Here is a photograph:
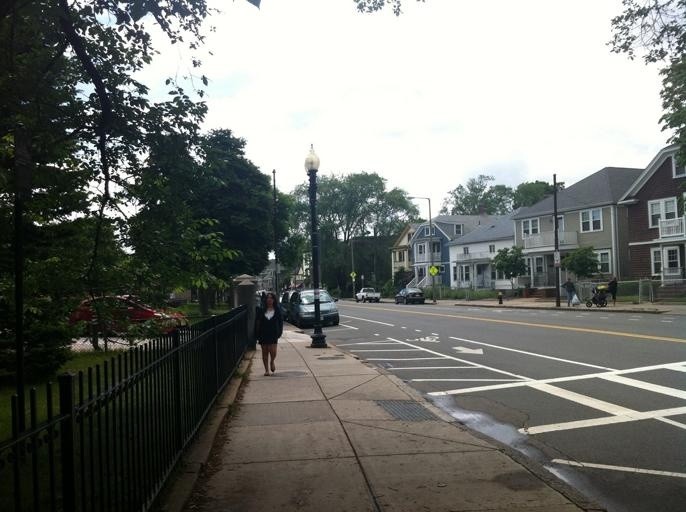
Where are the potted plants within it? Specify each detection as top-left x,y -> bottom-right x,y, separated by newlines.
522,282 -> 533,298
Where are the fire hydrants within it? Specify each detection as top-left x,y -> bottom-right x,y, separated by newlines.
497,292 -> 503,304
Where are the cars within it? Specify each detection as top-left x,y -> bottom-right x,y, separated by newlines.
68,294 -> 187,337
394,288 -> 425,305
166,292 -> 187,307
279,287 -> 340,329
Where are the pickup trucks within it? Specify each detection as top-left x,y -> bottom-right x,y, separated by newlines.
356,287 -> 381,303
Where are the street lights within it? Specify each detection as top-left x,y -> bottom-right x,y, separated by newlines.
301,142 -> 331,349
335,229 -> 357,299
406,196 -> 437,302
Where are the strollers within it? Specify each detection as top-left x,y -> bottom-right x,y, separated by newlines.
584,285 -> 610,307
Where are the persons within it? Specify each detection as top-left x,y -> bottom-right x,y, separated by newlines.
260,292 -> 266,308
608,278 -> 617,307
560,278 -> 576,307
256,293 -> 283,376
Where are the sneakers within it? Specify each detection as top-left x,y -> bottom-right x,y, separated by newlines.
270,361 -> 275,372
264,371 -> 269,376
